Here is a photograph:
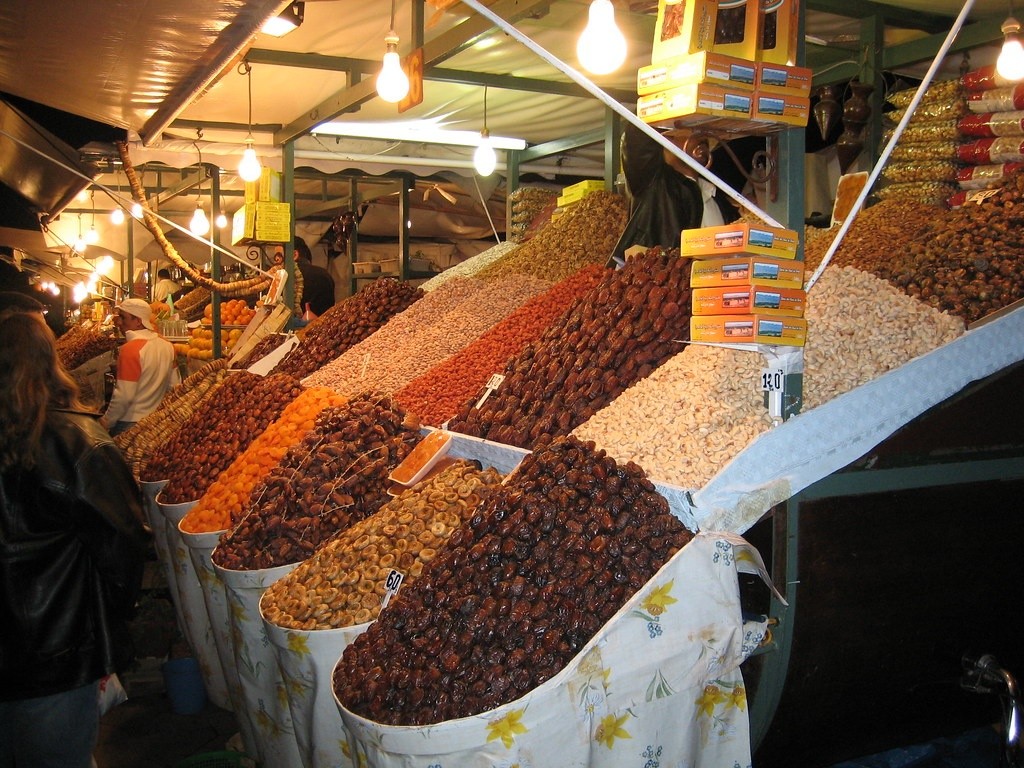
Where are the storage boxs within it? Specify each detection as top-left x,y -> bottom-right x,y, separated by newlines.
231,169 -> 291,246
680,223 -> 809,347
636,0 -> 813,137
380,258 -> 432,272
352,262 -> 381,274
557,180 -> 605,211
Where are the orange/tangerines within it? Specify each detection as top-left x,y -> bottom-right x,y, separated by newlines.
171,299 -> 263,359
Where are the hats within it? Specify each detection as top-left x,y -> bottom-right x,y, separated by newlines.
114,298 -> 154,331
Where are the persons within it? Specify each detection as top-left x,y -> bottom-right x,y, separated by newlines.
1,292 -> 155,768
151,268 -> 182,303
275,236 -> 335,317
98,299 -> 176,439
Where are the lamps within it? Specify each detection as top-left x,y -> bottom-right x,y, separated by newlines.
260,1 -> 305,38
310,122 -> 530,151
